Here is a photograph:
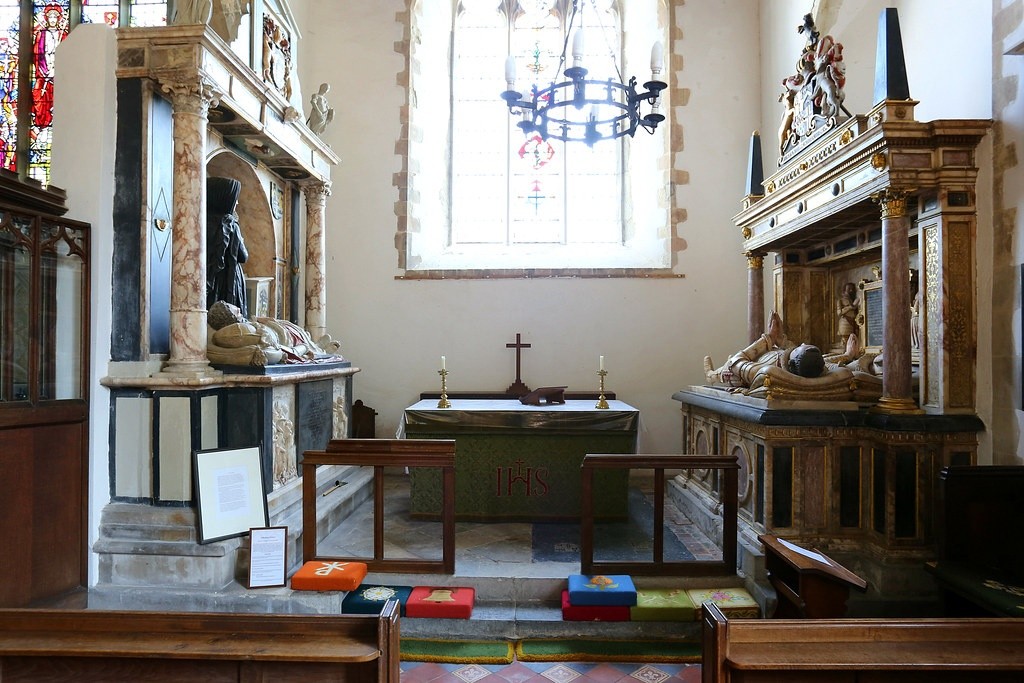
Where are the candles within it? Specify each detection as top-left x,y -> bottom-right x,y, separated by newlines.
600,355 -> 604,370
441,354 -> 445,369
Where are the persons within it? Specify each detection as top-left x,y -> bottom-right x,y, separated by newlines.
206,177 -> 249,318
824,334 -> 883,375
911,291 -> 919,347
837,283 -> 860,345
703,310 -> 826,387
208,300 -> 340,364
310,83 -> 334,135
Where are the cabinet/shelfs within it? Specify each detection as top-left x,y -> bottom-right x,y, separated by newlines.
0,201 -> 91,605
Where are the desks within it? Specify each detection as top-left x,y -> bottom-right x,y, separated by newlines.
394,399 -> 639,523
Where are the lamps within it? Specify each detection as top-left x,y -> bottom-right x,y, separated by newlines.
501,0 -> 668,143
